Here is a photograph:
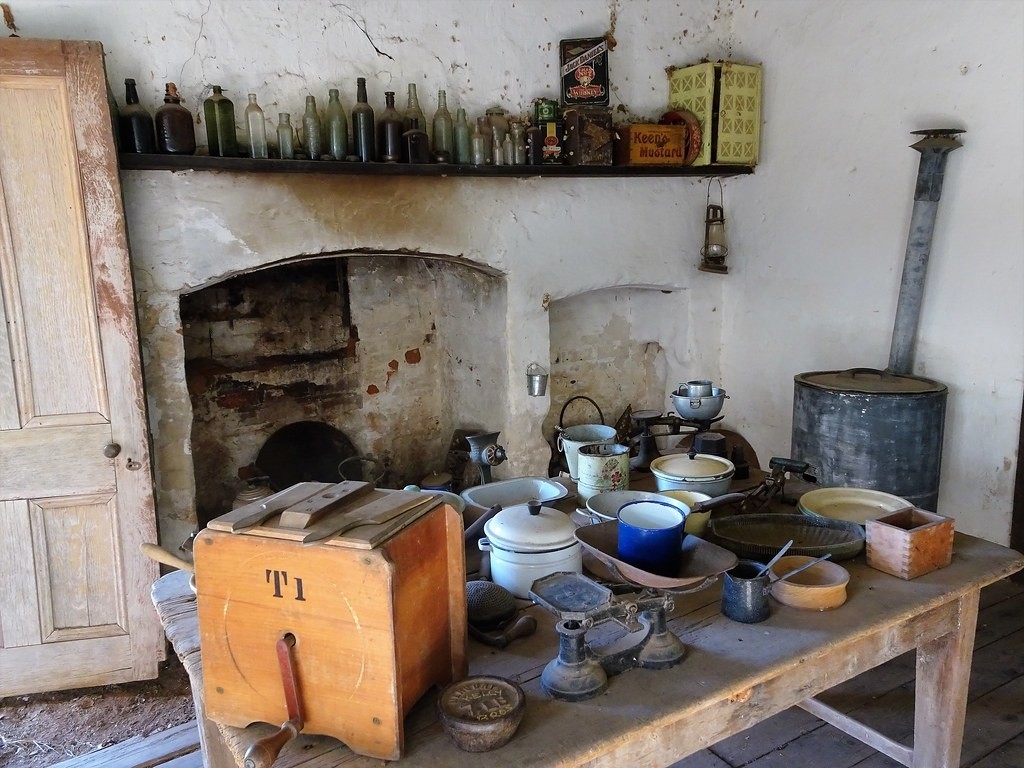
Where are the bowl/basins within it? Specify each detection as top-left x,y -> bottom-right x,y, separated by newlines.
669,389 -> 730,419
649,451 -> 736,497
656,490 -> 712,538
477,498 -> 582,600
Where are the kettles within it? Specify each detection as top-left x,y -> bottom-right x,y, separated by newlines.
233,478 -> 275,512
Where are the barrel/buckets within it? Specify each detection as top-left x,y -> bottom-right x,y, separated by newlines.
554,423 -> 617,483
524,362 -> 549,397
791,369 -> 948,514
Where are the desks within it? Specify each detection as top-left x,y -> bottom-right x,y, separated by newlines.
149,461 -> 1024,768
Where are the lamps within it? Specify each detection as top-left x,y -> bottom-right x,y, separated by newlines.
697,177 -> 728,275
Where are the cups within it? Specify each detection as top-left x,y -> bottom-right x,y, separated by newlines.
577,444 -> 630,500
616,501 -> 685,572
677,380 -> 713,396
720,561 -> 774,623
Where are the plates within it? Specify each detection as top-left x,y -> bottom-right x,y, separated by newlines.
585,491 -> 691,520
797,487 -> 915,525
708,513 -> 867,564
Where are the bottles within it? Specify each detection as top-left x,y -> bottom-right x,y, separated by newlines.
117,78 -> 542,164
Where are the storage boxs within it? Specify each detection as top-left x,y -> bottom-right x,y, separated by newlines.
616,125 -> 685,164
865,505 -> 955,581
